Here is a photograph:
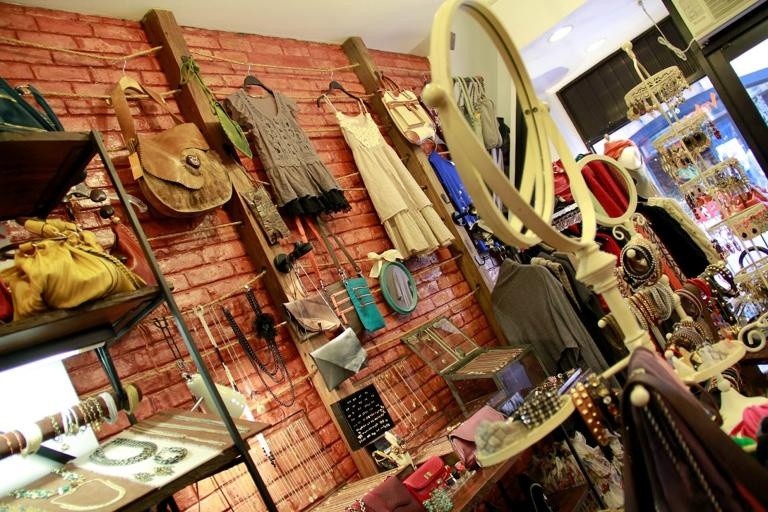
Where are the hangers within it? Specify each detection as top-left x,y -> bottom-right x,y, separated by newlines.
432,131 -> 450,155
491,209 -> 584,274
240,62 -> 277,97
316,69 -> 369,108
105,57 -> 167,107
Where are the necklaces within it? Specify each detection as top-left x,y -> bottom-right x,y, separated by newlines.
51,478 -> 125,509
185,304 -> 256,397
154,316 -> 188,374
93,439 -> 155,465
219,287 -> 298,407
377,359 -> 440,430
458,348 -> 522,373
139,325 -> 157,349
228,417 -> 334,511
142,418 -> 246,435
12,470 -> 83,501
130,425 -> 227,448
153,410 -> 250,432
320,468 -> 398,511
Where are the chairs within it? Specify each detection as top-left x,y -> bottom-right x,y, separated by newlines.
401,316 -> 550,418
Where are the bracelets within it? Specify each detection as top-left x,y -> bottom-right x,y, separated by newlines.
98,391 -> 120,425
120,383 -> 140,414
23,426 -> 43,457
48,398 -> 103,437
10,430 -> 24,453
599,236 -> 741,359
155,448 -> 190,465
0,435 -> 16,454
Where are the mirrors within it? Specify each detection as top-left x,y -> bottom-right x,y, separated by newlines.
570,154 -> 638,228
380,260 -> 418,314
421,0 -> 598,247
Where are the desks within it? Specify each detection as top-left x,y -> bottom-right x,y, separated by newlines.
445,367 -> 612,512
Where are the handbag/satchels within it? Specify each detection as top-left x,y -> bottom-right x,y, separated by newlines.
452,76 -> 485,149
90,190 -> 159,287
457,74 -> 503,149
0,219 -> 145,322
349,476 -> 427,512
403,456 -> 454,509
111,76 -> 233,216
380,75 -> 436,145
233,155 -> 293,246
182,58 -> 253,158
281,255 -> 340,344
286,255 -> 368,392
448,405 -> 507,469
0,76 -> 63,133
316,213 -> 386,333
